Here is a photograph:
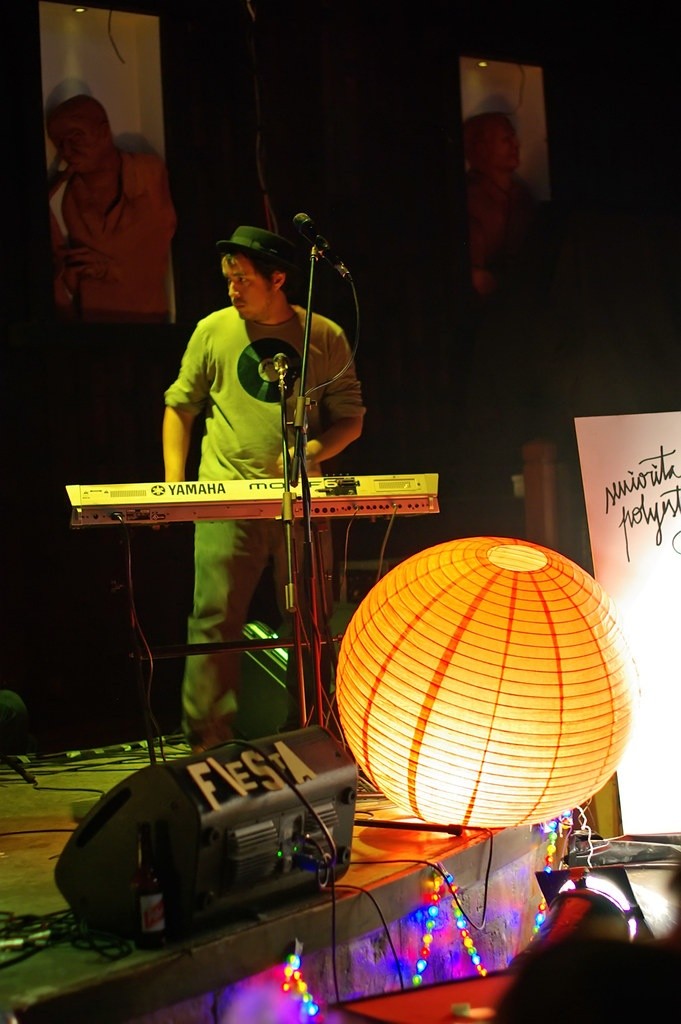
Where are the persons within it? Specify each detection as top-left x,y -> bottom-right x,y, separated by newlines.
464,111 -> 531,296
49,94 -> 175,326
152,225 -> 365,753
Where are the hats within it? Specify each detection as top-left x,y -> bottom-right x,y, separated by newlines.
216,226 -> 310,280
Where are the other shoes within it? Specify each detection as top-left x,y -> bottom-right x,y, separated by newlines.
185,724 -> 234,755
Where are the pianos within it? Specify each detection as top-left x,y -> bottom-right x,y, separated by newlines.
65,471 -> 442,527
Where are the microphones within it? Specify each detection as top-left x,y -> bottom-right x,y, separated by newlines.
272,353 -> 291,385
292,213 -> 354,282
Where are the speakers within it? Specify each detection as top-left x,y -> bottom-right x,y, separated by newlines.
53,725 -> 357,952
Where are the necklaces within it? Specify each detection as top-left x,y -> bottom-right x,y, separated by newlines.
489,178 -> 507,195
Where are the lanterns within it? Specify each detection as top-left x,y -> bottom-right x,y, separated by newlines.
336,534 -> 640,828
